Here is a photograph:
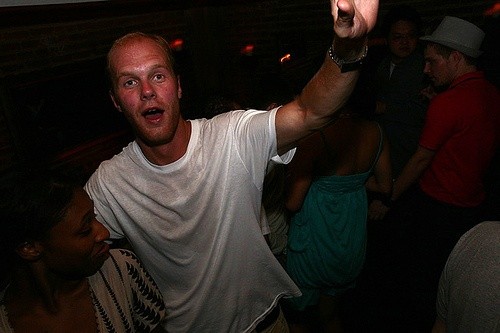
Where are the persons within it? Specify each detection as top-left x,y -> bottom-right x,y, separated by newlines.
83,0 -> 381,333
438,220 -> 500,333
214,11 -> 500,305
0,168 -> 168,333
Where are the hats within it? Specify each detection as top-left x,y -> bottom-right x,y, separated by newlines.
417,15 -> 486,58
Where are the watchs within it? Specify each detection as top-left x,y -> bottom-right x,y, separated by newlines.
328,44 -> 368,72
